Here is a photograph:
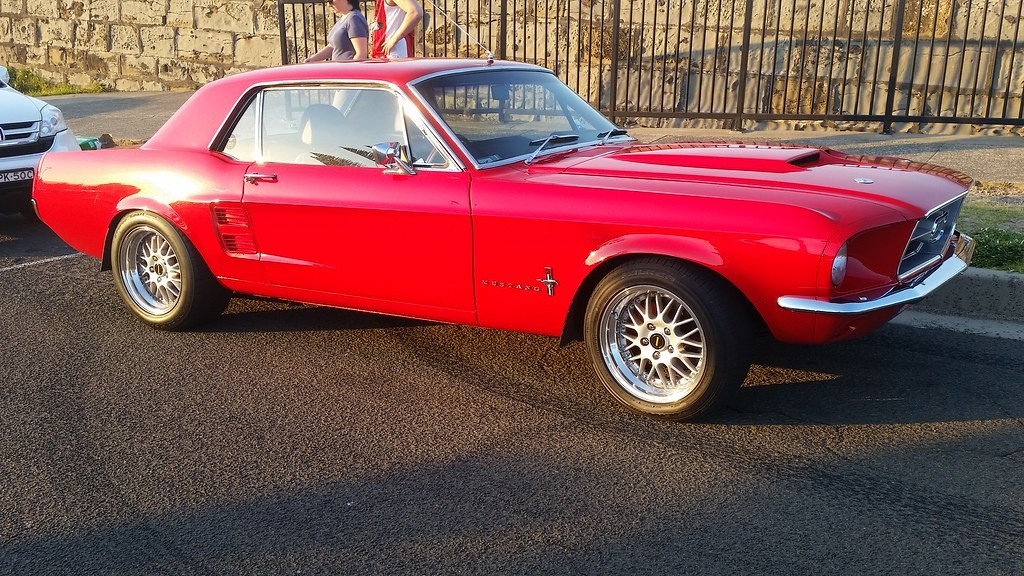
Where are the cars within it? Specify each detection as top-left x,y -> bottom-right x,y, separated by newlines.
0,67 -> 82,220
29,58 -> 978,423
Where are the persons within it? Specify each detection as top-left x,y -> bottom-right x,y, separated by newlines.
372,0 -> 423,64
301,0 -> 368,115
